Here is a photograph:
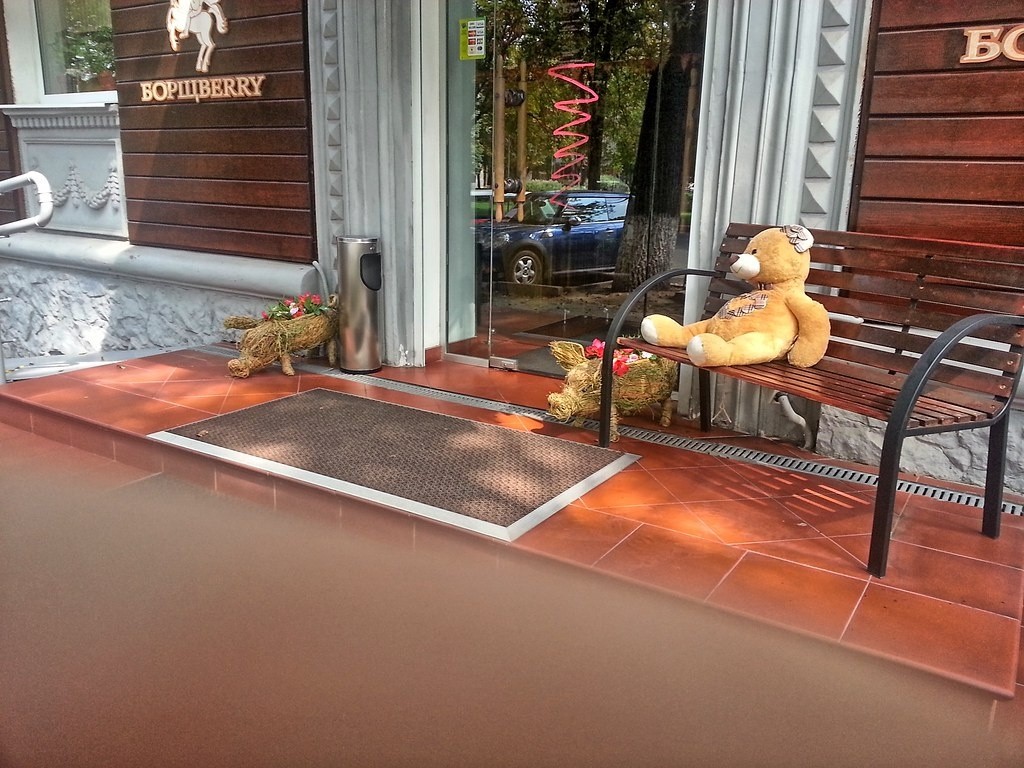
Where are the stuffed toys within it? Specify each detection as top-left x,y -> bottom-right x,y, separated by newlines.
639,224 -> 832,369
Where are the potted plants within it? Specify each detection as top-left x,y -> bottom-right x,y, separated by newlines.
58,25 -> 117,91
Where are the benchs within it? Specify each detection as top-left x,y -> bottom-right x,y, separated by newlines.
599,222 -> 1024,578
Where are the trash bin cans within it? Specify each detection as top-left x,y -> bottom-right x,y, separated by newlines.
335,235 -> 383,374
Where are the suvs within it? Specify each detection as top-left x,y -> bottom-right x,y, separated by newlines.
476,189 -> 631,296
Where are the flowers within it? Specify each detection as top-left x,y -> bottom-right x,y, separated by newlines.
262,291 -> 331,328
586,337 -> 659,376
